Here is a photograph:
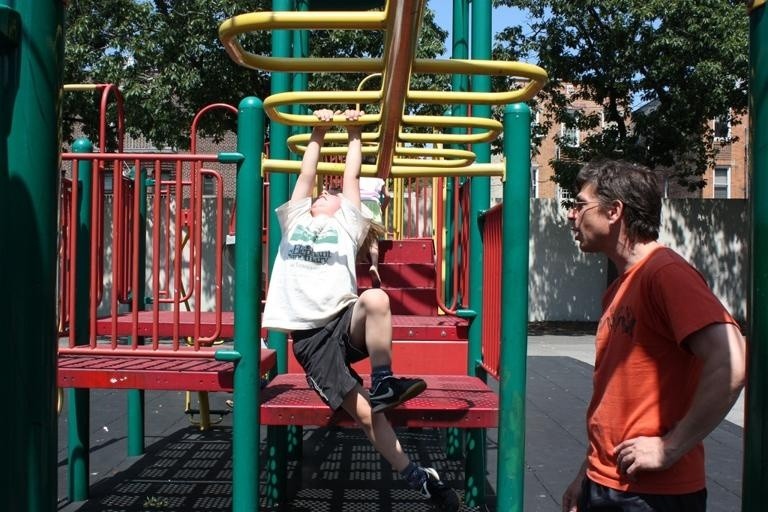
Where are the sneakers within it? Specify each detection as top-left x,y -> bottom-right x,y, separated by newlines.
367,372 -> 428,415
416,465 -> 462,512
368,264 -> 382,288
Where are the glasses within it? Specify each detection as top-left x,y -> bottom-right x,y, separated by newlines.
571,199 -> 613,213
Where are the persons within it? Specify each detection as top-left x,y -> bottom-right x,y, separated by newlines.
260,108 -> 460,512
562,155 -> 746,512
359,155 -> 391,289
